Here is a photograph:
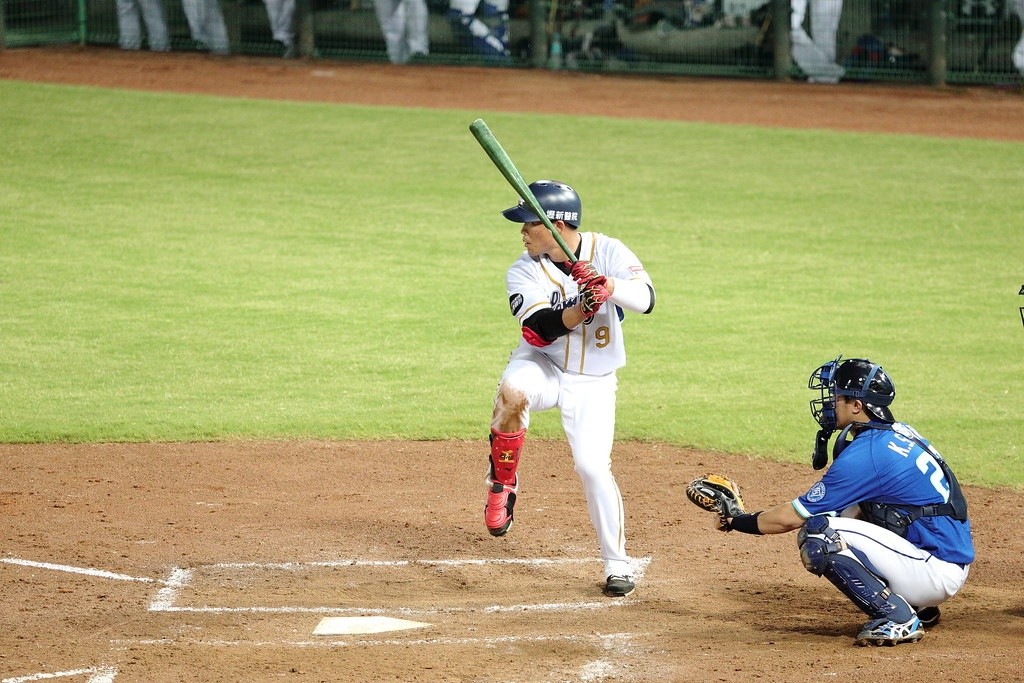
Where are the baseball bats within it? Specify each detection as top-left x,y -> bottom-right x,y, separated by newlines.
469,118 -> 580,264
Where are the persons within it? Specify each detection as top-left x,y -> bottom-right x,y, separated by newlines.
484,179 -> 655,597
790,0 -> 845,85
111,0 -> 513,65
686,358 -> 975,647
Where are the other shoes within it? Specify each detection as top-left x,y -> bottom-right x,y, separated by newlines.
409,45 -> 430,64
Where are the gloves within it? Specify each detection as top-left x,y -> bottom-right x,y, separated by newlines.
575,286 -> 608,325
564,261 -> 601,285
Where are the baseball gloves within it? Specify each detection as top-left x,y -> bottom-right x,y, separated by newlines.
685,472 -> 746,533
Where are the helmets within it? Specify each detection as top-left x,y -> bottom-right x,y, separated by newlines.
831,358 -> 897,424
501,180 -> 583,228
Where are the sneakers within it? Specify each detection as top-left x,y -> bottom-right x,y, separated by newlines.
606,575 -> 636,596
856,611 -> 926,647
912,605 -> 940,625
483,465 -> 519,537
